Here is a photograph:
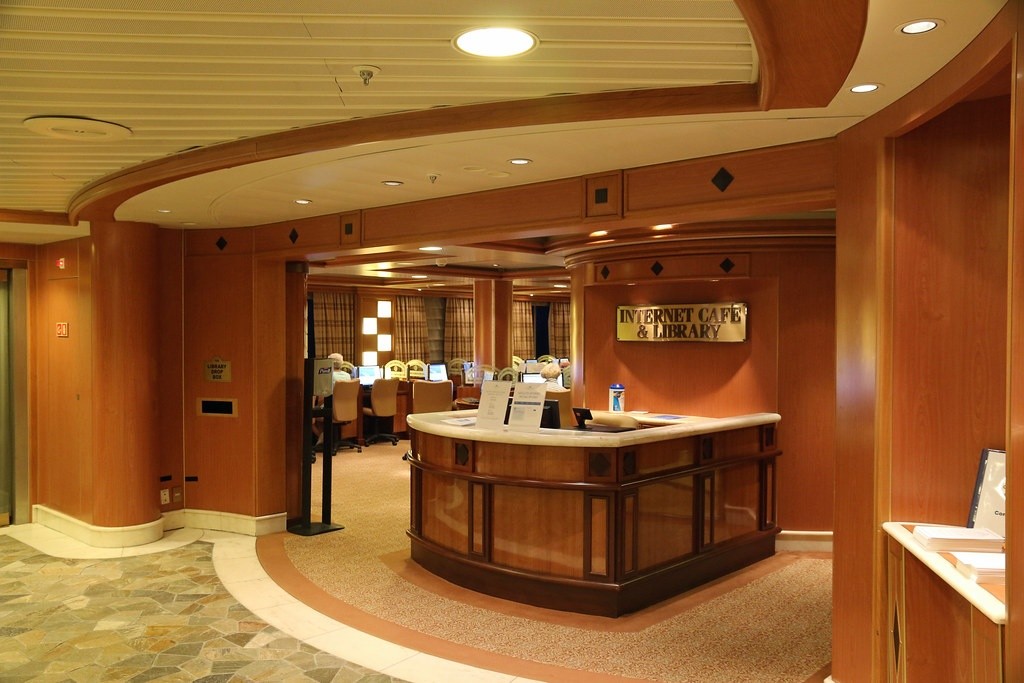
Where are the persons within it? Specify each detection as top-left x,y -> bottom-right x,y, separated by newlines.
311,354 -> 351,443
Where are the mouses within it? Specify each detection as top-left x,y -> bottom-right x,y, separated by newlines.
474,401 -> 479,404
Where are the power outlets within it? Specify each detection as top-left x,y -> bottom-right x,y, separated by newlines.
160,488 -> 170,504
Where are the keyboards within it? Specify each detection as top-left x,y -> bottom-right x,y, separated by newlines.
462,397 -> 478,403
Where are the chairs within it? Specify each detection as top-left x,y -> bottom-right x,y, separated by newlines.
307,363 -> 570,464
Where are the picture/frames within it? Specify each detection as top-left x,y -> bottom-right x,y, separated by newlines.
966,448 -> 1006,539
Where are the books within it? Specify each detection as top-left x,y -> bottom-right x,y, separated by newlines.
951,552 -> 1005,584
913,526 -> 1005,551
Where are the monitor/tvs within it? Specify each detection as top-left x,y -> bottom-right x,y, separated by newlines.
480,370 -> 495,394
521,372 -> 564,387
525,357 -> 568,365
357,365 -> 381,386
430,363 -> 449,380
462,361 -> 474,386
504,397 -> 561,429
